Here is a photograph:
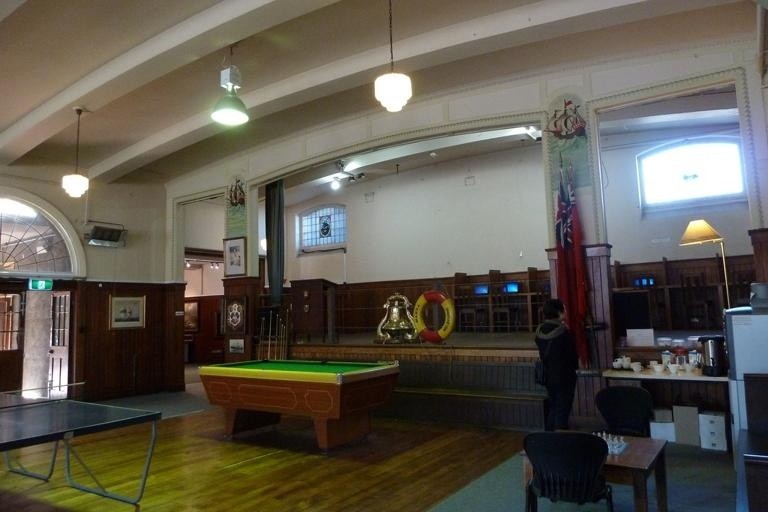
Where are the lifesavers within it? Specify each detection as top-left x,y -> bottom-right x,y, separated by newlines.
413,290 -> 455,343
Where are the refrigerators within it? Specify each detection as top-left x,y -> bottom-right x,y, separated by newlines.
724,305 -> 768,471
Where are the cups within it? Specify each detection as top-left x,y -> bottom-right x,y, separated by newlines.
613,357 -> 695,374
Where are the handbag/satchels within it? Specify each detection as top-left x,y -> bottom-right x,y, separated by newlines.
535,360 -> 545,385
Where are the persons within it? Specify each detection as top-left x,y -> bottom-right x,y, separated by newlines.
534,299 -> 579,432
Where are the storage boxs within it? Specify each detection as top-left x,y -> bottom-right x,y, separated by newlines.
650,407 -> 729,451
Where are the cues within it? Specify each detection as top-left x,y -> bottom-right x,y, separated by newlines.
258,309 -> 289,360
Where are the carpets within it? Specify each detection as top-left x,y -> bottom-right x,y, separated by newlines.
430,452 -> 737,511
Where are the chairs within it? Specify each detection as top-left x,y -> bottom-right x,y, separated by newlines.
522,385 -> 653,512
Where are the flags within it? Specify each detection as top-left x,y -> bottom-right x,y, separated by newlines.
557,169 -> 575,331
566,173 -> 591,369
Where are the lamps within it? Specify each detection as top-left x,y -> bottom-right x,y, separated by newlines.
373,1 -> 413,114
679,219 -> 732,310
61,108 -> 89,199
210,42 -> 251,128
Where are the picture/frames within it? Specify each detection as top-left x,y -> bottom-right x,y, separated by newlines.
222,236 -> 248,277
107,294 -> 146,331
227,337 -> 245,354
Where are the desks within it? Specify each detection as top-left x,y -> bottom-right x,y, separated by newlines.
613,282 -> 723,332
0,393 -> 162,504
452,291 -> 551,333
519,428 -> 668,512
197,359 -> 401,454
